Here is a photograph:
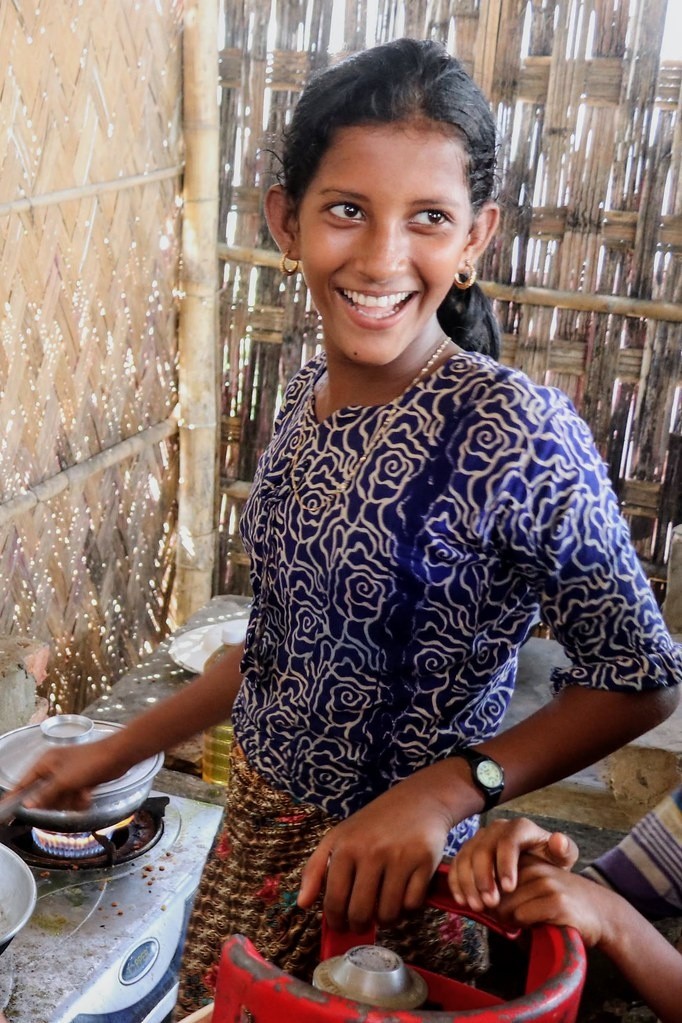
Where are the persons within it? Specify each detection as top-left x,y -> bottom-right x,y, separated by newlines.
448,781 -> 682,1023
0,38 -> 682,1023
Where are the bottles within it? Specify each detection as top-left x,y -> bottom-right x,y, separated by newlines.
202,626 -> 246,806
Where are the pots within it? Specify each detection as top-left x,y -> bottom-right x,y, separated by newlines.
0,843 -> 38,955
0,714 -> 165,833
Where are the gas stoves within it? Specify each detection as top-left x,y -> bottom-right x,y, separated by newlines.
0,790 -> 224,1023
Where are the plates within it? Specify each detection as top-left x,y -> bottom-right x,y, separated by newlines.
170,618 -> 249,674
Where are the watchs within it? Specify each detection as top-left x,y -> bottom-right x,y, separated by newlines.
446,748 -> 506,815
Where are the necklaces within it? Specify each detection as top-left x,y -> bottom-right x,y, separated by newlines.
291,336 -> 451,511
